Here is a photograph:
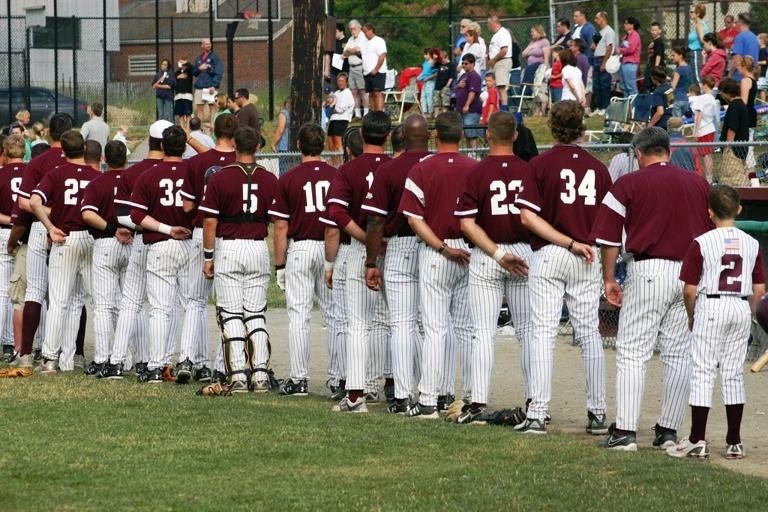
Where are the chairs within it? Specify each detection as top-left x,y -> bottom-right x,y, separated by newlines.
585,96 -> 639,144
381,67 -> 426,124
508,64 -> 539,117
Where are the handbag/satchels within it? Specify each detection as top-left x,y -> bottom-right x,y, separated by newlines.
605,53 -> 621,74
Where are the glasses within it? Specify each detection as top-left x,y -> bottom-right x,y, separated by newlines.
462,62 -> 468,65
235,96 -> 242,100
690,10 -> 696,13
459,24 -> 465,28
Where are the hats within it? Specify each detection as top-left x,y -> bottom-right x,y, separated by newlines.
149,118 -> 174,139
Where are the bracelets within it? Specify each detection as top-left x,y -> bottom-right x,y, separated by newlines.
438,242 -> 448,254
365,262 -> 377,269
567,239 -> 576,254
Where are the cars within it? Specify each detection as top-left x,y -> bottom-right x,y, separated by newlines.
0,86 -> 90,128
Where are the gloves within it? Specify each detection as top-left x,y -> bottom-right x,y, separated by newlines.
274,265 -> 285,291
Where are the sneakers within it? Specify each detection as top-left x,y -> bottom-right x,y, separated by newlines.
0,353 -> 12,363
722,442 -> 745,459
135,363 -> 146,375
230,380 -> 249,393
649,423 -> 676,450
437,395 -> 455,412
513,417 -> 547,434
137,369 -> 163,383
278,378 -> 309,396
7,354 -> 33,368
585,410 -> 609,435
384,398 -> 410,415
365,392 -> 380,405
404,403 -> 439,418
212,369 -> 226,383
33,360 -> 57,373
94,364 -> 124,380
33,354 -> 42,363
84,362 -> 105,377
332,396 -> 369,413
329,387 -> 347,401
175,362 -> 191,385
545,413 -> 552,424
253,380 -> 269,393
593,423 -> 637,450
193,367 -> 211,381
665,435 -> 710,459
455,408 -> 486,425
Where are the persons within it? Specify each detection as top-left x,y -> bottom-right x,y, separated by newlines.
0,135 -> 27,359
7,143 -> 51,364
570,8 -> 597,107
270,95 -> 290,176
456,30 -> 482,81
521,24 -> 551,116
728,12 -> 759,80
449,108 -> 553,426
212,88 -> 260,146
646,21 -> 665,88
552,17 -> 572,49
756,33 -> 768,105
558,48 -> 587,106
360,113 -> 435,415
416,48 -> 438,114
666,116 -> 702,175
9,112 -> 73,369
94,118 -> 210,380
481,73 -> 500,140
646,67 -> 674,131
671,44 -> 691,117
80,141 -> 133,375
321,73 -> 355,167
736,55 -> 757,175
616,16 -> 641,99
80,102 -> 110,163
487,15 -> 513,112
454,54 -> 483,124
10,102 -> 48,162
360,23 -> 387,112
430,50 -> 453,118
151,58 -> 176,123
174,52 -> 193,119
718,15 -> 738,50
173,112 -> 237,384
569,38 -> 590,88
699,31 -> 727,153
686,3 -> 706,95
331,22 -> 349,92
589,126 -> 716,452
591,11 -> 616,107
513,99 -> 613,435
322,131 -> 362,401
688,77 -> 716,184
343,20 -> 368,120
666,185 -> 765,460
130,126 -> 212,384
30,130 -> 103,375
268,124 -> 338,395
608,132 -> 640,253
197,126 -> 278,393
506,28 -> 521,106
57,140 -> 105,372
454,18 -> 473,72
326,109 -> 395,414
549,47 -> 564,103
397,113 -> 480,418
194,39 -> 224,130
718,78 -> 749,187
111,125 -> 130,155
468,22 -> 487,80
